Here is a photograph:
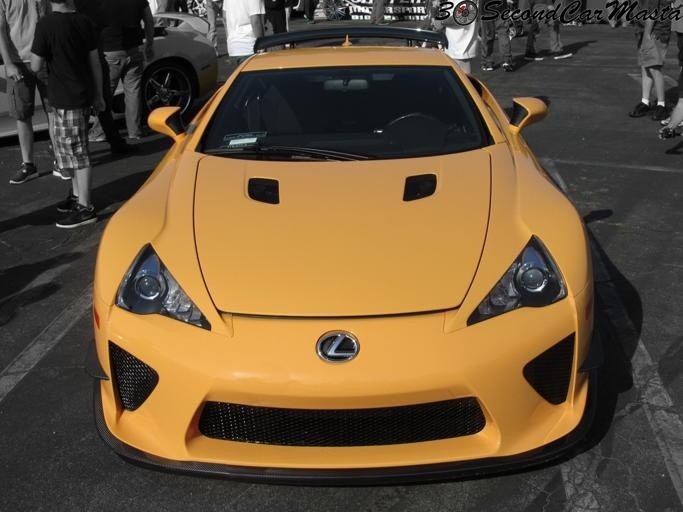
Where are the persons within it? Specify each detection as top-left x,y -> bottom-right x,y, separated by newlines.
629,0 -> 683,140
140,0 -> 188,29
30,1 -> 106,229
207,0 -> 319,71
607,0 -> 630,29
426,0 -> 573,74
369,0 -> 390,26
0,0 -> 155,184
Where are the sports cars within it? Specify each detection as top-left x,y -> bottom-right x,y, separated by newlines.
92,26 -> 596,486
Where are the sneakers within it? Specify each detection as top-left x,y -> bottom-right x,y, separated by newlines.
480,62 -> 512,72
87,133 -> 107,142
554,50 -> 573,60
52,161 -> 72,180
56,195 -> 80,212
629,100 -> 653,118
658,117 -> 677,139
524,52 -> 544,61
129,127 -> 147,139
9,163 -> 39,185
55,202 -> 97,229
652,102 -> 668,121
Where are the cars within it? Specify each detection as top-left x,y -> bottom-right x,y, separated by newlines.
0,1 -> 524,140
